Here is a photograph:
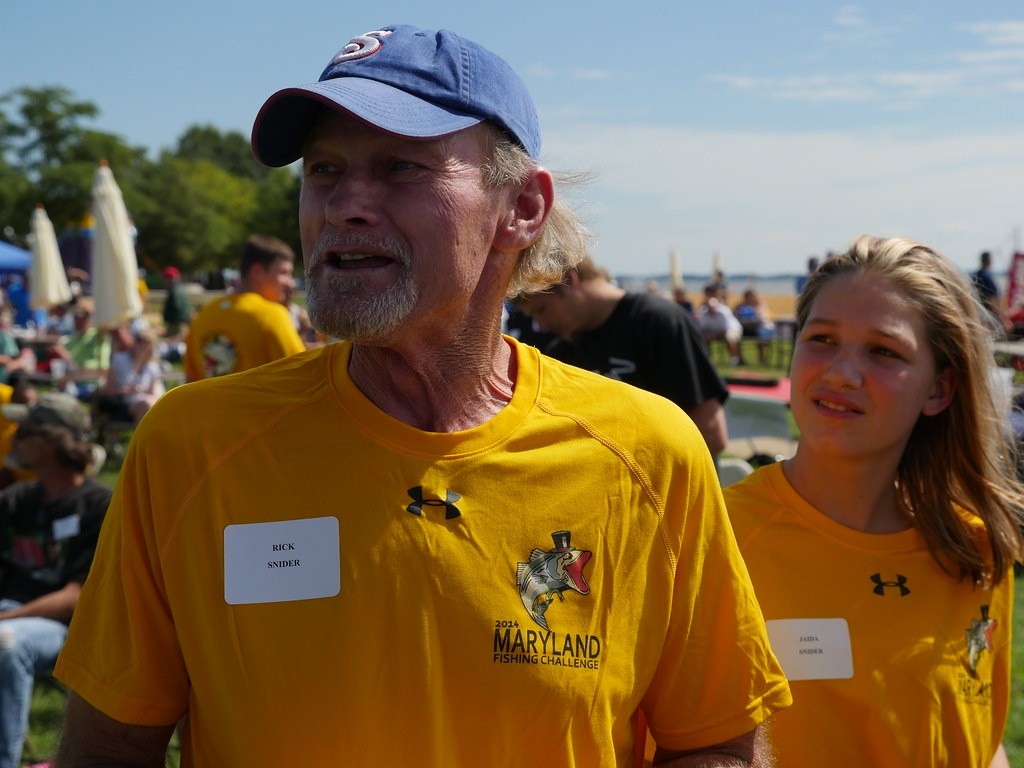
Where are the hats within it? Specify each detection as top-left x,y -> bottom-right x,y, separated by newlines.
0,394 -> 90,433
250,24 -> 541,168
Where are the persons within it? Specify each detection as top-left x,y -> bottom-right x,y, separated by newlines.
1,234 -> 356,458
504,232 -> 728,478
630,235 -> 1024,768
0,394 -> 115,768
50,21 -> 795,768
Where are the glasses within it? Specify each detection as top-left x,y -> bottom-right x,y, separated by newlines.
10,422 -> 49,442
